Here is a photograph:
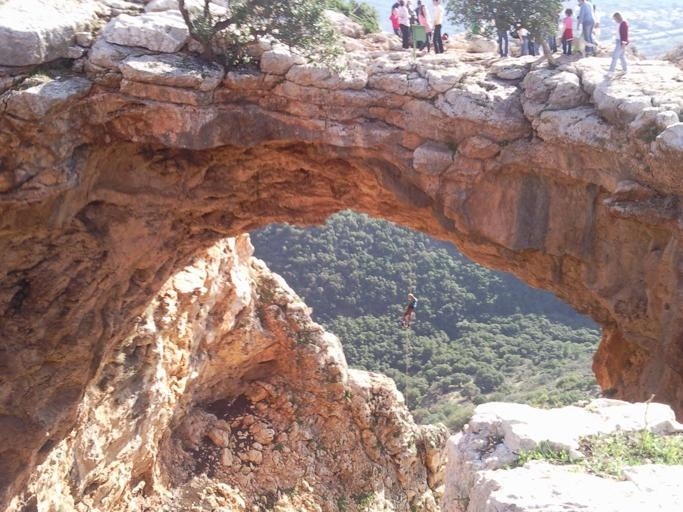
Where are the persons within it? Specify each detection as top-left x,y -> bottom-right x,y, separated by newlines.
495,1 -> 601,60
389,1 -> 448,55
403,291 -> 419,328
606,12 -> 631,76
